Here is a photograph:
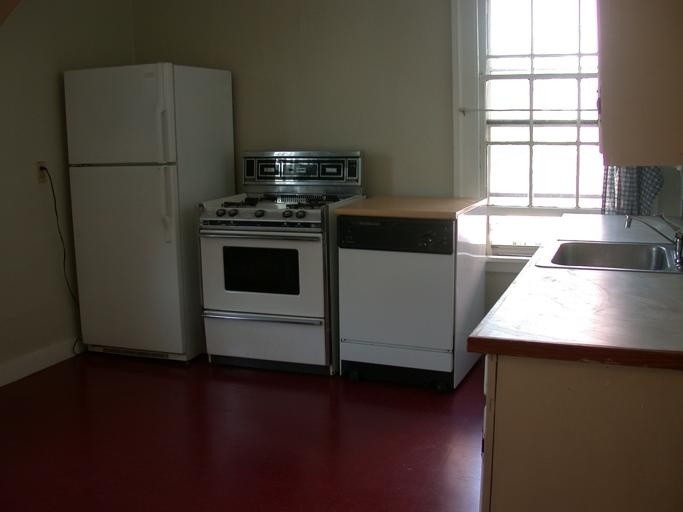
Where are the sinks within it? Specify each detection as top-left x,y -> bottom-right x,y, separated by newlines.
534,238 -> 680,273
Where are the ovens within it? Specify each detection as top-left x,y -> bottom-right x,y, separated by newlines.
198,229 -> 333,378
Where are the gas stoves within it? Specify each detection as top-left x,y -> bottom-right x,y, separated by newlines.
201,193 -> 348,223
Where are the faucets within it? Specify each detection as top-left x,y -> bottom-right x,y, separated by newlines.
622,213 -> 683,271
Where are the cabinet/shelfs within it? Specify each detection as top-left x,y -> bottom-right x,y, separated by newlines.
595,0 -> 682,168
336,214 -> 488,391
480,355 -> 681,511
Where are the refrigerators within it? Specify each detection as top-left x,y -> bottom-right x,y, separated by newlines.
58,64 -> 236,366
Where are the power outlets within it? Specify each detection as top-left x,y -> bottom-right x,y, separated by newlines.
34,161 -> 49,185
